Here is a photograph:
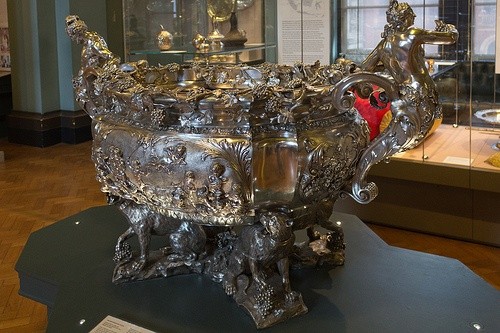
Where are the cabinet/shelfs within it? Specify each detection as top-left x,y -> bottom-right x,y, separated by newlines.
122,0 -> 279,72
